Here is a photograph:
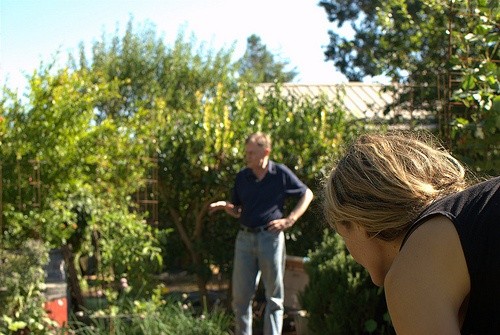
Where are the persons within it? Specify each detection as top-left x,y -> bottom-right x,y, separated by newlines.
323,131 -> 500,335
209,131 -> 313,335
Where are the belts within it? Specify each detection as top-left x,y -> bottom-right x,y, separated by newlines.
239,225 -> 272,234
